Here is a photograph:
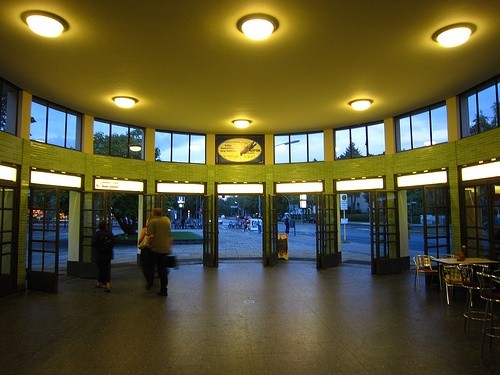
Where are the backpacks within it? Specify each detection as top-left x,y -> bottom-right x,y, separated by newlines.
98,232 -> 113,254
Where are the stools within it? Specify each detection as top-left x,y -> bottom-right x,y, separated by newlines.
457,261 -> 500,359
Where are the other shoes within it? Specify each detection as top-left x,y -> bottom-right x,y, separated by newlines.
159,291 -> 166,296
145,283 -> 151,288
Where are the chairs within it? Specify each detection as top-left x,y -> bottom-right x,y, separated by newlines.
412,253 -> 474,306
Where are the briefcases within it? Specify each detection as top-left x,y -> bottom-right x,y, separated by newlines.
165,254 -> 176,268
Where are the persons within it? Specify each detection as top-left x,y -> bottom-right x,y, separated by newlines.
258,217 -> 262,234
139,218 -> 156,288
94,220 -> 115,291
285,214 -> 289,233
243,217 -> 249,231
144,208 -> 173,296
174,218 -> 185,229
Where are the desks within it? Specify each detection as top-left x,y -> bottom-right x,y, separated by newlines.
429,257 -> 498,305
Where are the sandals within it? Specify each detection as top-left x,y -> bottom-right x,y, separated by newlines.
95,284 -> 103,288
105,287 -> 110,292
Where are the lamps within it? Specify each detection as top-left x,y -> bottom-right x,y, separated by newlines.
232,119 -> 253,128
431,20 -> 478,48
349,98 -> 374,112
237,12 -> 279,43
130,145 -> 142,151
112,95 -> 141,107
20,9 -> 69,37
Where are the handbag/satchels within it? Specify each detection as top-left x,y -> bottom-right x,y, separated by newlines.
138,227 -> 150,249
